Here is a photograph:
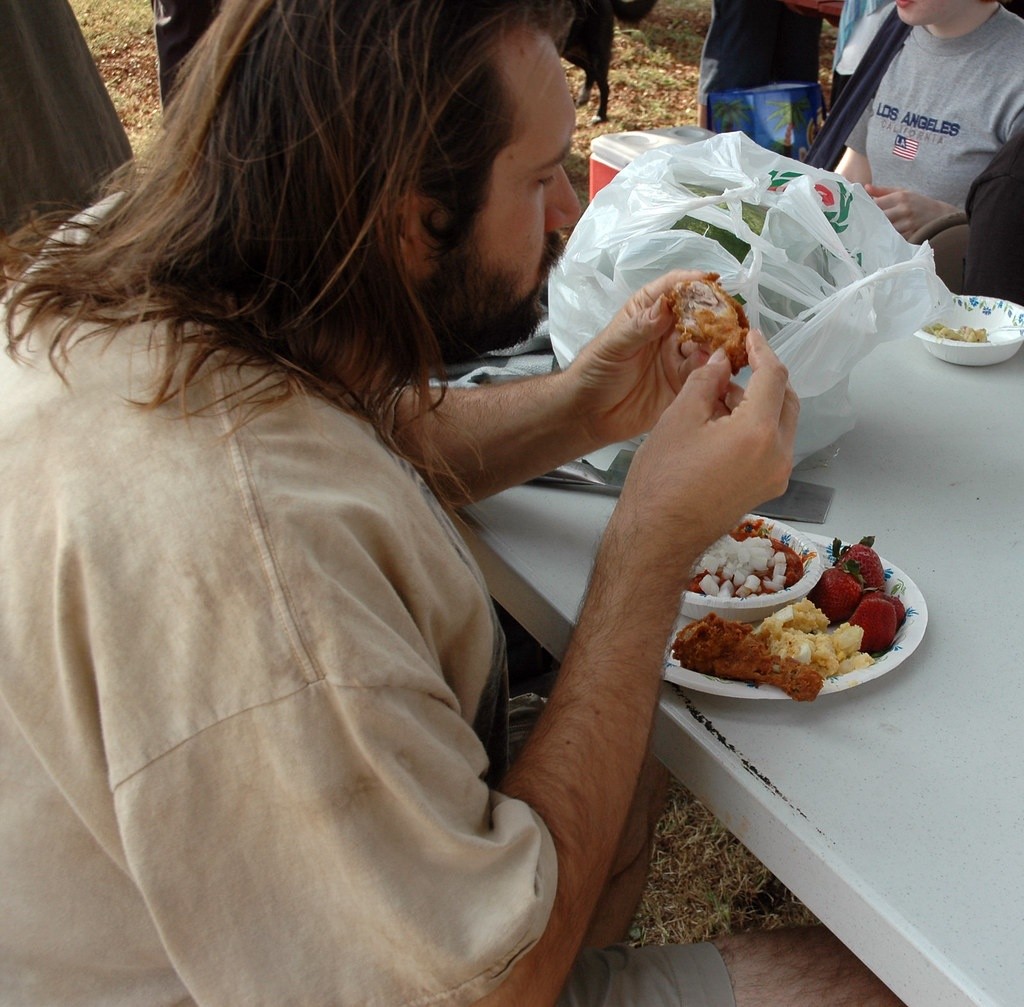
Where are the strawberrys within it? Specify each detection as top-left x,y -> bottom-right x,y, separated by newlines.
806,544 -> 906,654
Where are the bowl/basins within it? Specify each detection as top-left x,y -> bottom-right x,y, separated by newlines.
678,513 -> 822,625
913,295 -> 1024,366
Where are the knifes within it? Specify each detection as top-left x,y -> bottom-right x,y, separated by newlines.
527,448 -> 837,525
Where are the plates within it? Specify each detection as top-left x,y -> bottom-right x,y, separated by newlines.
663,532 -> 928,700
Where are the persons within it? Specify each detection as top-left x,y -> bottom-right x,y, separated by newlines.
697,0 -> 1024,306
0,0 -> 802,1007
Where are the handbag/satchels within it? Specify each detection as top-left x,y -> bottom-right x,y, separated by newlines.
547,131 -> 953,468
706,81 -> 828,163
